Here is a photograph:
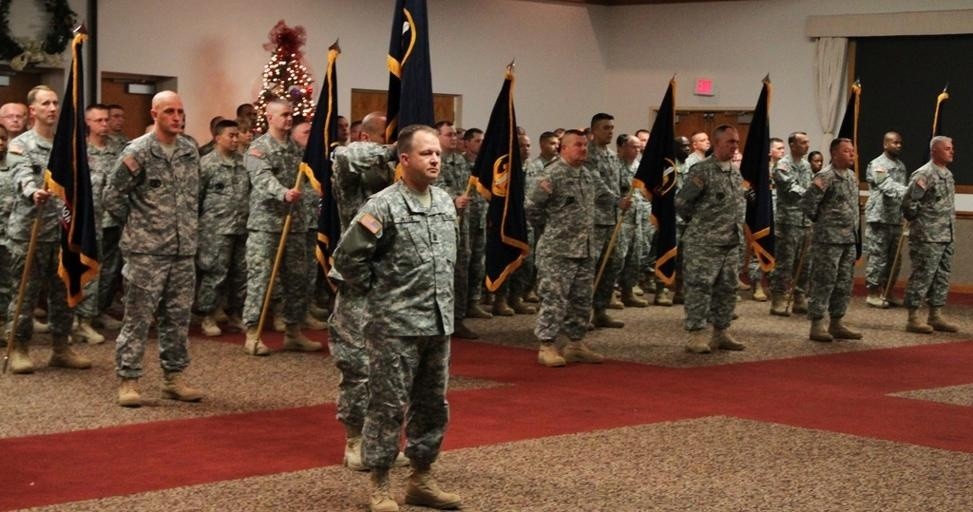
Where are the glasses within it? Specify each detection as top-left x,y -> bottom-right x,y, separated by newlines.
110,114 -> 125,118
88,118 -> 109,122
2,114 -> 23,118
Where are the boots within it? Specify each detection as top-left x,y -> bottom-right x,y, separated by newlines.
598,289 -> 672,327
684,328 -> 711,354
710,327 -> 745,351
117,376 -> 144,406
159,367 -> 202,403
10,309 -> 122,372
562,340 -> 602,361
826,317 -> 863,339
200,305 -> 330,354
537,341 -> 567,366
467,286 -> 539,317
741,282 -> 810,314
867,289 -> 961,332
808,317 -> 834,342
342,438 -> 465,510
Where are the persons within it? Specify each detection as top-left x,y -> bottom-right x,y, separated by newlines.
533,113 -> 711,369
440,119 -> 535,336
329,108 -> 413,475
1,86 -> 130,375
103,89 -> 211,408
188,98 -> 361,354
736,133 -> 961,344
326,121 -> 471,511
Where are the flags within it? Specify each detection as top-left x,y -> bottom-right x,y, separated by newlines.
732,77 -> 780,270
926,93 -> 950,138
630,77 -> 683,290
42,36 -> 100,298
832,82 -> 864,265
465,72 -> 533,295
388,0 -> 437,140
300,49 -> 349,294
